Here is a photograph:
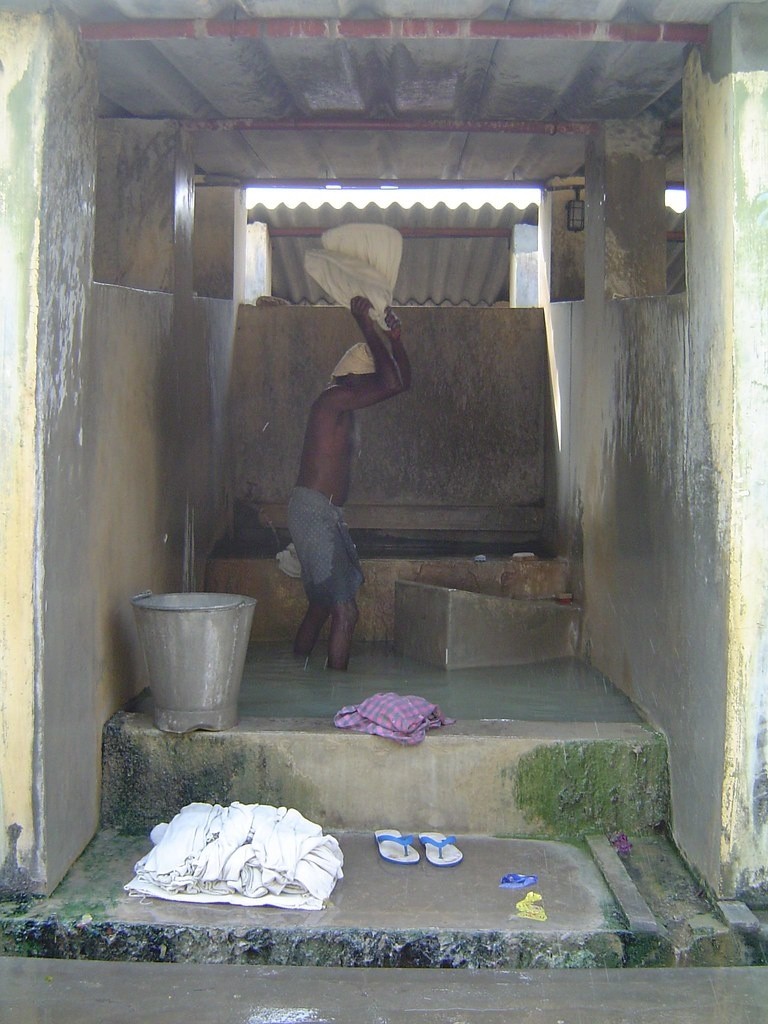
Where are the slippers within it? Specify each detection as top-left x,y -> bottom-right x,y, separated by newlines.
374,828 -> 422,865
418,832 -> 464,868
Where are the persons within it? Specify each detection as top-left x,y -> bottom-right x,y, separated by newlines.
288,296 -> 412,670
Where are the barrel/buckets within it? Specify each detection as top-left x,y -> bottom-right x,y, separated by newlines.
129,590 -> 257,735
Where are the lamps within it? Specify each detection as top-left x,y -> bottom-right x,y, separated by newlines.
563,188 -> 591,234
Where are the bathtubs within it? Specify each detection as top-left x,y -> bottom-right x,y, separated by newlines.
204,528 -> 562,644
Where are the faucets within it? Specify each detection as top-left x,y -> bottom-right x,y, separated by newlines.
243,481 -> 264,514
